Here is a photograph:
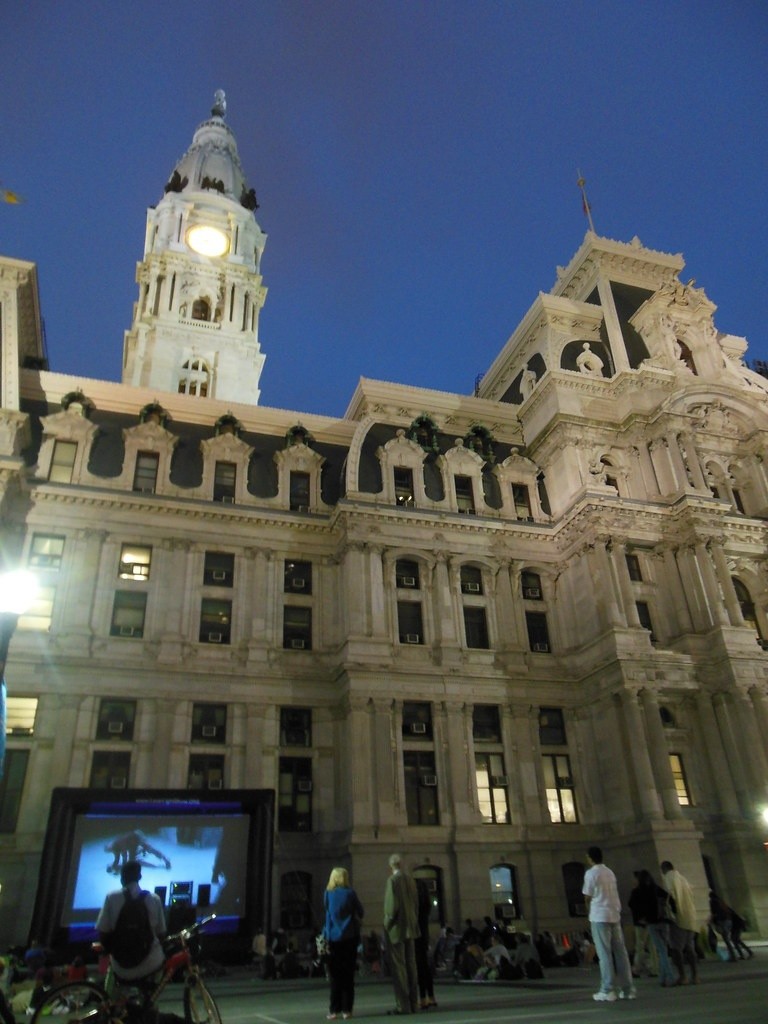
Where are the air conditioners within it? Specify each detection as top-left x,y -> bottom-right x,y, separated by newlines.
119,625 -> 135,637
402,577 -> 550,736
208,632 -> 222,643
207,778 -> 223,790
201,726 -> 216,736
421,775 -> 437,786
298,780 -> 312,792
141,486 -> 535,522
292,578 -> 305,590
110,776 -> 127,789
574,903 -> 587,915
291,639 -> 304,650
213,570 -> 226,582
425,880 -> 436,892
289,914 -> 304,928
107,721 -> 123,734
494,776 -> 509,787
498,906 -> 516,918
560,776 -> 577,787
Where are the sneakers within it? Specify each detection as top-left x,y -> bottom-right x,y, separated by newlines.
621,989 -> 636,1000
593,990 -> 617,1002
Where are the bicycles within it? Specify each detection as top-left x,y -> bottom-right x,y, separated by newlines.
27,913 -> 224,1024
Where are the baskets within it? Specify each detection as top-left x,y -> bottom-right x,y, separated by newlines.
160,933 -> 210,971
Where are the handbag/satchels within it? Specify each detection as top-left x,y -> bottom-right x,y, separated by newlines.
497,955 -> 512,979
474,964 -> 488,980
664,905 -> 677,923
551,955 -> 560,967
487,968 -> 499,980
316,934 -> 331,957
525,958 -> 544,979
512,956 -> 523,979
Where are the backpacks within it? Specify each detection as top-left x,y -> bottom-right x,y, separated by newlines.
110,888 -> 149,968
312,959 -> 326,978
461,951 -> 478,974
568,951 -> 579,966
364,936 -> 380,962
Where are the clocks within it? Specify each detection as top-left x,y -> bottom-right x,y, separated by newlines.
186,222 -> 231,259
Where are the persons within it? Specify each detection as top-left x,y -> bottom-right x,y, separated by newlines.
433,916 -> 599,984
105,829 -> 170,876
0,941 -> 88,1024
705,890 -> 757,962
384,855 -> 437,1016
703,321 -> 724,369
169,168 -> 181,191
659,314 -> 682,360
95,861 -> 167,1012
211,816 -> 245,911
251,929 -> 390,980
582,846 -> 635,1001
324,868 -> 364,1019
241,182 -> 260,213
628,859 -> 699,987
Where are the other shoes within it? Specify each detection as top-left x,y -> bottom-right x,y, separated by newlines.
342,1011 -> 352,1020
418,1002 -> 438,1010
689,975 -> 701,984
388,1004 -> 410,1015
672,976 -> 689,986
326,1010 -> 337,1020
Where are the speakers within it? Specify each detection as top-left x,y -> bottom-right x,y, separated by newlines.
197,885 -> 211,906
154,886 -> 166,906
